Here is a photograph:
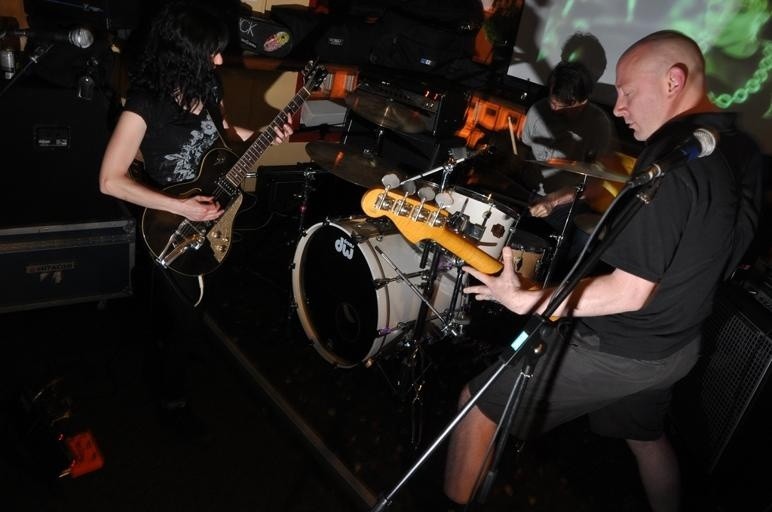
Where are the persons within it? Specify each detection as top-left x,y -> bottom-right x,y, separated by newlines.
520,62 -> 611,284
100,9 -> 294,467
435,29 -> 745,511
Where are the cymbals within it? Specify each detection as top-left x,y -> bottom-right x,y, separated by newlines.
305,139 -> 416,195
528,154 -> 632,188
344,88 -> 428,136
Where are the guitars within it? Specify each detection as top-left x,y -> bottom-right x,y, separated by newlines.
359,184 -> 564,328
137,60 -> 329,280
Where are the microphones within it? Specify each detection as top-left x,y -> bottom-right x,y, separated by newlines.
629,128 -> 715,187
17,27 -> 94,48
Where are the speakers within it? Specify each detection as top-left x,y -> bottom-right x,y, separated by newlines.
0,83 -> 114,227
669,280 -> 772,478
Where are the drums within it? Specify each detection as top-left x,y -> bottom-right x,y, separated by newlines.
499,230 -> 552,284
429,183 -> 523,266
288,214 -> 475,374
